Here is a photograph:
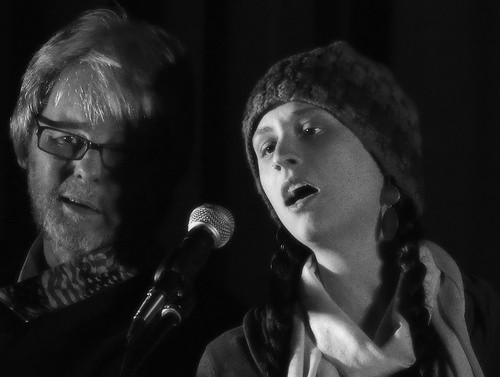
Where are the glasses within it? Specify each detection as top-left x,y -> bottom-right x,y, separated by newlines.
32,114 -> 144,175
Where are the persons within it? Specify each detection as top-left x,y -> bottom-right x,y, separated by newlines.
195,41 -> 500,377
0,8 -> 236,377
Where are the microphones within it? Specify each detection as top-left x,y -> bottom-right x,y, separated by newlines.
125,203 -> 236,345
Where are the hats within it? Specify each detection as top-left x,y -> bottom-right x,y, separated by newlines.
242,40 -> 424,226
